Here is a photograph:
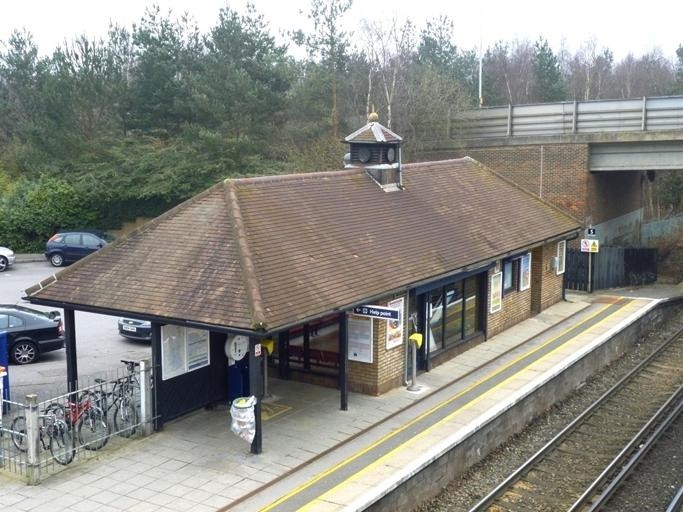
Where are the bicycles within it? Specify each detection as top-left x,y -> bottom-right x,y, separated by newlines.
9,357 -> 152,465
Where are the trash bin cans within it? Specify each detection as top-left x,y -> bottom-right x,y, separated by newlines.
230,397 -> 257,445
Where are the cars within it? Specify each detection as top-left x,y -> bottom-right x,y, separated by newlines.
0,231 -> 151,364
